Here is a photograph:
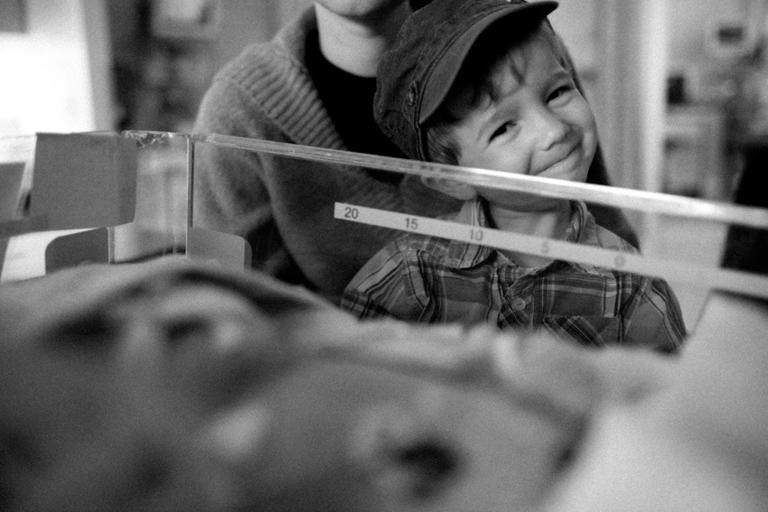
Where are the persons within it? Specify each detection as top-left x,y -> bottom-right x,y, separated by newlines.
337,1 -> 685,361
185,1 -> 643,311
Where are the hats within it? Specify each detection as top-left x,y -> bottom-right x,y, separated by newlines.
372,0 -> 560,164
